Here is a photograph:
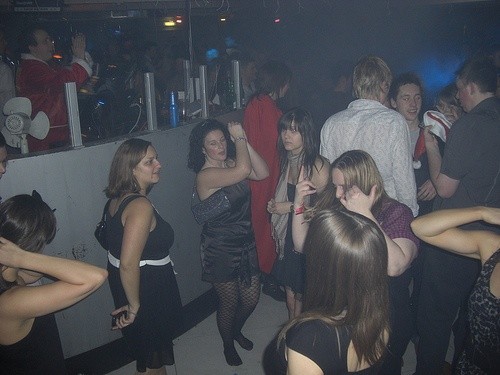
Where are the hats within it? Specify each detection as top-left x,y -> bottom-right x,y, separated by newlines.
412,110 -> 453,169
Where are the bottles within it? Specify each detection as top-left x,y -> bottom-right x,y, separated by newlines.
169,90 -> 180,128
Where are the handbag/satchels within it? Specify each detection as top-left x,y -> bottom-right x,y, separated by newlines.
191,188 -> 230,223
94,201 -> 109,250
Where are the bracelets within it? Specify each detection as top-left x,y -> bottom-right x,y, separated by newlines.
126,304 -> 139,316
233,136 -> 246,144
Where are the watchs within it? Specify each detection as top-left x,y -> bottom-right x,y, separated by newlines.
290,201 -> 294,213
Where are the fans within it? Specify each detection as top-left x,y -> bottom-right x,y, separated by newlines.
0,97 -> 51,155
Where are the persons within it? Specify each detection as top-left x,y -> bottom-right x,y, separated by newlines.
83,33 -> 275,138
389,73 -> 436,215
0,61 -> 15,153
0,192 -> 110,375
308,54 -> 359,131
410,206 -> 500,375
15,27 -> 94,150
413,53 -> 500,375
318,55 -> 418,218
414,80 -> 463,216
262,208 -> 404,375
188,118 -> 271,366
242,58 -> 293,301
266,106 -> 333,321
94,139 -> 183,375
0,131 -> 7,182
293,148 -> 420,360
